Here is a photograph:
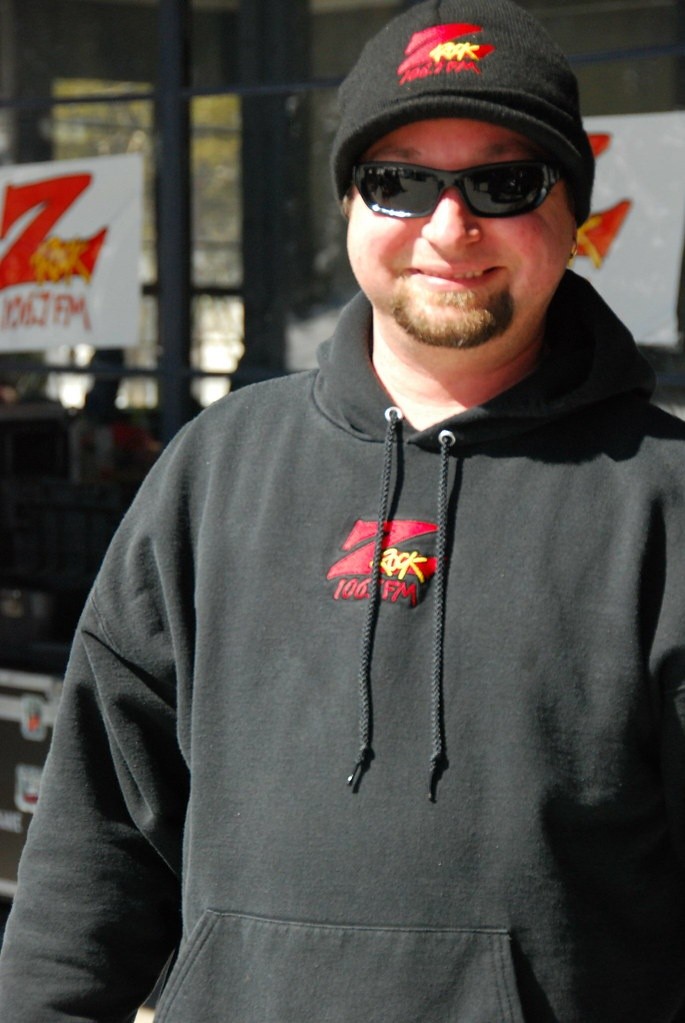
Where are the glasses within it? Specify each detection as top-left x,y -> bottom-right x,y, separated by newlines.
351,155 -> 566,217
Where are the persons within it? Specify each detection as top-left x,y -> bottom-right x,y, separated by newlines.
0,0 -> 685,1023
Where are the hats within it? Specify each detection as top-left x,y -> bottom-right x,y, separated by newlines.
331,0 -> 595,228
